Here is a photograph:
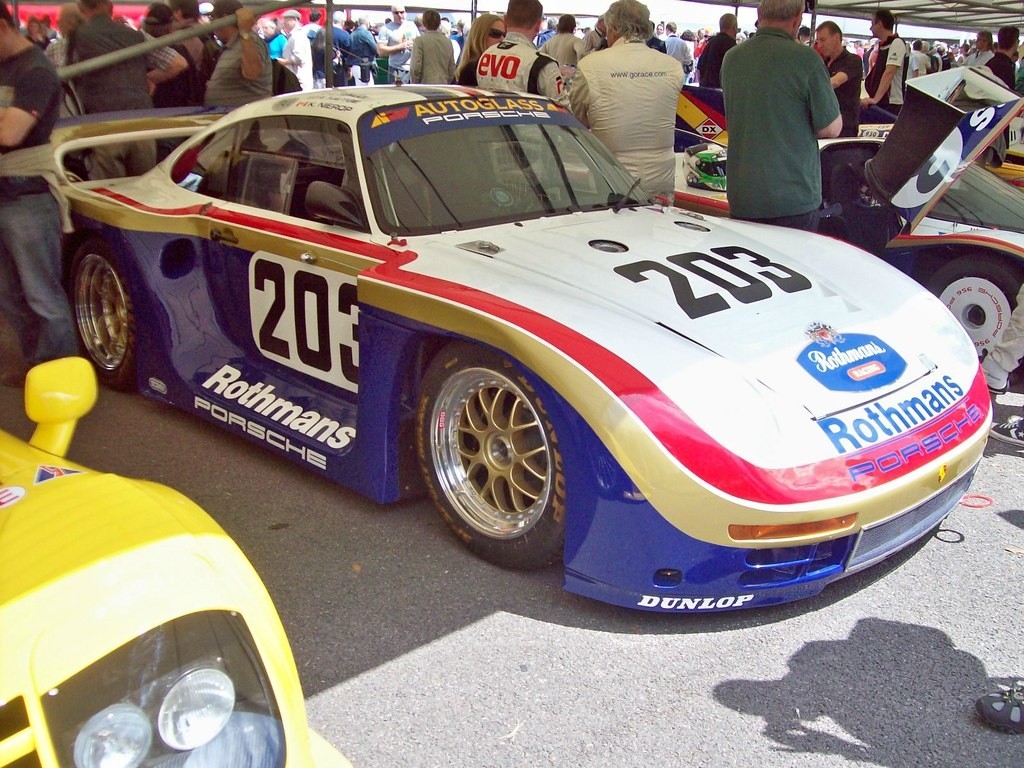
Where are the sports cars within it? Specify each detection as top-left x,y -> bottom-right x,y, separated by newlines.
51,78 -> 994,614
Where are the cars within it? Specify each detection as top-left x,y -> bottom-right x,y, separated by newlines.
671,64 -> 1023,366
1,358 -> 312,768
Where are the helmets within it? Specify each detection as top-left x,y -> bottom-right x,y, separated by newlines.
682,142 -> 727,192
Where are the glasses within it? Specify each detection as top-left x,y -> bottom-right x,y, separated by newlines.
208,15 -> 216,21
541,15 -> 547,22
488,28 -> 506,39
392,11 -> 406,14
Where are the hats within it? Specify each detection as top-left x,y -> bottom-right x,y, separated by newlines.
144,3 -> 174,26
333,11 -> 346,22
280,9 -> 301,19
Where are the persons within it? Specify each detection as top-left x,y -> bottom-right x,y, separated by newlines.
0,0 -> 1024,449
201,140 -> 309,213
975,688 -> 1024,735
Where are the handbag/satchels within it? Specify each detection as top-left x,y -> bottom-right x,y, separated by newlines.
270,58 -> 303,97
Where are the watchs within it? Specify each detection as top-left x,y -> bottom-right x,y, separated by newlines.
239,31 -> 252,40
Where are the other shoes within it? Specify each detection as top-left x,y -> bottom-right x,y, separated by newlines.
975,686 -> 1024,735
0,357 -> 38,390
